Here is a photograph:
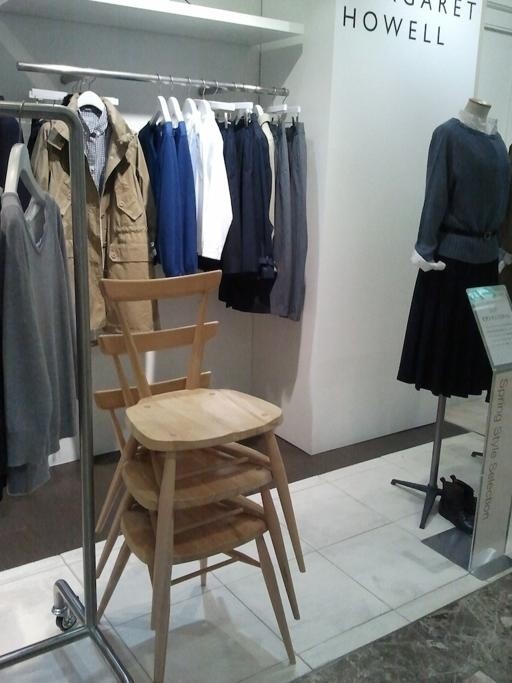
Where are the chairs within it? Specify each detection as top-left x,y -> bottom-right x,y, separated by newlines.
95,270 -> 305,683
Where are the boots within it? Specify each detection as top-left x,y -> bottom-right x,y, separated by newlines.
437,475 -> 478,535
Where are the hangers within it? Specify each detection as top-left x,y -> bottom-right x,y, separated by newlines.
30,69 -> 303,159
1,98 -> 65,247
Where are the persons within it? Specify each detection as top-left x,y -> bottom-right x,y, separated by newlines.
395,96 -> 512,403
